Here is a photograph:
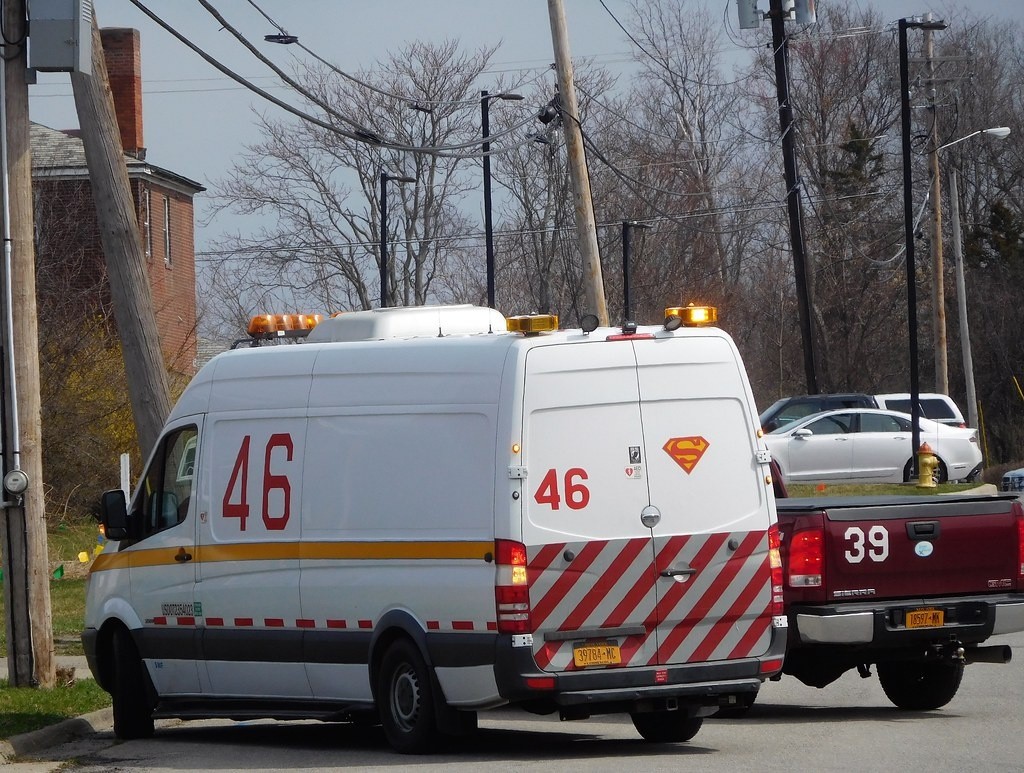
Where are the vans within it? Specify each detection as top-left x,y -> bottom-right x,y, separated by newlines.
81,300 -> 789,758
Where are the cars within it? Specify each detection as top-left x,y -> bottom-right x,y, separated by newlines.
756,409 -> 983,488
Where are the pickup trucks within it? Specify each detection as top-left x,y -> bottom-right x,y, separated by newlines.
706,453 -> 1024,717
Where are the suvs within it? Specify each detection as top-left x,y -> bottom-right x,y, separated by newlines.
875,392 -> 965,427
758,393 -> 880,439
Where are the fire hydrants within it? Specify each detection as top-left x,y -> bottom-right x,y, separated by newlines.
915,441 -> 940,489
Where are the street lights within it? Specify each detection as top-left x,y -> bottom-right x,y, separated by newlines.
381,169 -> 415,309
622,219 -> 653,323
900,20 -> 946,488
478,88 -> 527,305
925,127 -> 1014,393
